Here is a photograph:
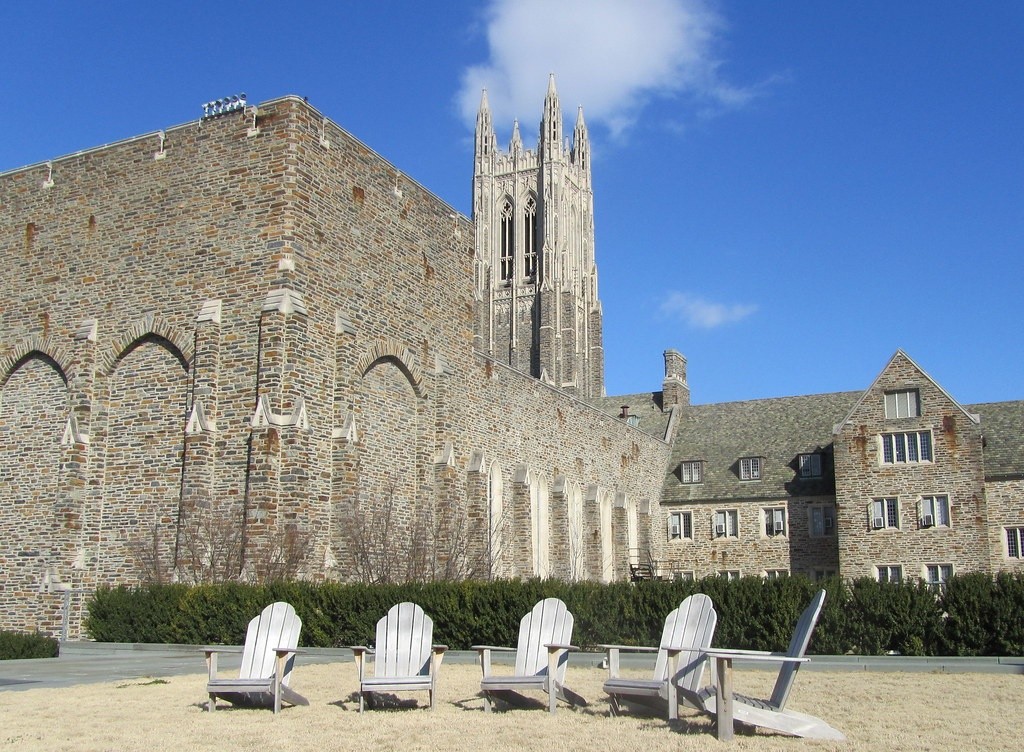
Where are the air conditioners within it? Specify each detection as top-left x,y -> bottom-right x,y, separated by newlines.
825,517 -> 833,527
924,514 -> 933,525
776,521 -> 784,530
672,525 -> 680,534
874,517 -> 884,528
717,524 -> 725,533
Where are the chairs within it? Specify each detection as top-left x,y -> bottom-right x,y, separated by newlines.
349,602 -> 448,713
471,597 -> 586,713
698,589 -> 845,740
597,594 -> 717,725
198,601 -> 309,714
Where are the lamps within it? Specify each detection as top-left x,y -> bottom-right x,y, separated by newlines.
202,93 -> 246,120
